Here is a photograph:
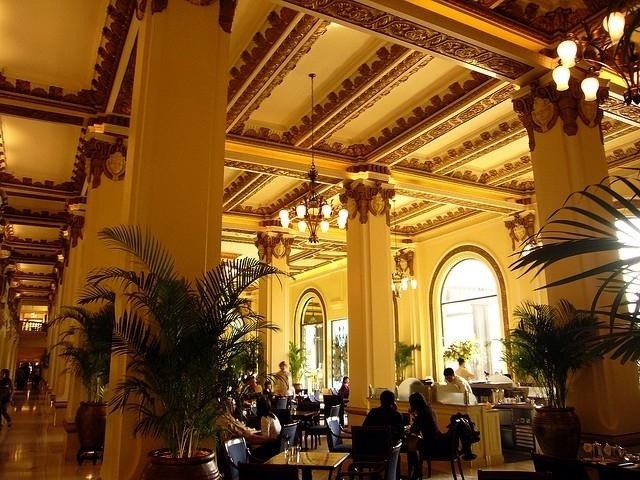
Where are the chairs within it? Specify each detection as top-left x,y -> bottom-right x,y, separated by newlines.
325,416 -> 354,473
350,424 -> 389,462
477,467 -> 544,478
337,441 -> 402,479
417,418 -> 467,478
472,377 -> 555,455
222,378 -> 348,448
225,438 -> 267,478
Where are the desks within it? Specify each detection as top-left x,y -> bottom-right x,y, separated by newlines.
263,451 -> 351,479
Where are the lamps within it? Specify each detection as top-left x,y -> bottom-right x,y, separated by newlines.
17,304 -> 43,334
549,1 -> 640,109
278,73 -> 350,245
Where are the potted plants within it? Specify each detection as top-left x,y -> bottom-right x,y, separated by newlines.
82,223 -> 295,480
501,297 -> 599,474
43,294 -> 117,466
287,342 -> 307,390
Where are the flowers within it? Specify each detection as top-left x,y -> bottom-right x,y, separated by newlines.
441,339 -> 480,363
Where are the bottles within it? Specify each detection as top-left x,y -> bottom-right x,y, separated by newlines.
289,445 -> 301,456
490,388 -> 505,404
592,441 -> 612,458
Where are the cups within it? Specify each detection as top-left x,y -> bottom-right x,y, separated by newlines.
613,447 -> 625,459
503,398 -> 517,404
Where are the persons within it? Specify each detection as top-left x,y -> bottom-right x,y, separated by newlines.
407,390 -> 444,480
337,376 -> 350,400
220,360 -> 295,442
0,368 -> 17,430
454,358 -> 477,382
443,367 -> 474,395
360,389 -> 405,480
14,359 -> 44,394
